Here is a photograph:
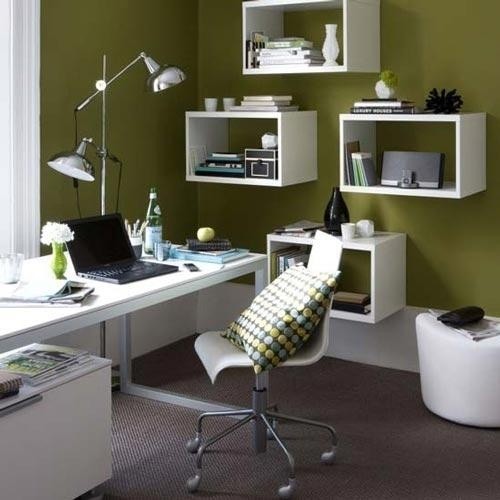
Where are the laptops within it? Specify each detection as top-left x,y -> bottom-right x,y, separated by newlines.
60,213 -> 178,284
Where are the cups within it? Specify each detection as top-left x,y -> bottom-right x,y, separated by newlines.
223,97 -> 235,111
154,240 -> 172,262
205,98 -> 218,111
340,224 -> 355,241
0,246 -> 25,285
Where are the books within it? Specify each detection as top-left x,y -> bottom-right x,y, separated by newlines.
1,341 -> 98,388
350,97 -> 416,114
344,139 -> 378,186
332,290 -> 371,315
0,371 -> 23,398
192,150 -> 245,179
2,273 -> 97,308
254,35 -> 323,66
270,245 -> 309,283
228,93 -> 300,113
172,238 -> 251,264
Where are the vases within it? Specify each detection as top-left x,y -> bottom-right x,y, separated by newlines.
50,240 -> 70,278
321,186 -> 351,238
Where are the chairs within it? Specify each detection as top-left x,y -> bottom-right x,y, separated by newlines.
184,226 -> 344,495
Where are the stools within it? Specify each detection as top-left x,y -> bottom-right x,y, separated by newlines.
414,308 -> 500,429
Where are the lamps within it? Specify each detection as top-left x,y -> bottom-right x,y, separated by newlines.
140,48 -> 189,94
46,137 -> 124,186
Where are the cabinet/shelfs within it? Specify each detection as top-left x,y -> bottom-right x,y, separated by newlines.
339,113 -> 487,200
0,342 -> 115,499
184,108 -> 320,189
242,0 -> 382,75
265,230 -> 408,327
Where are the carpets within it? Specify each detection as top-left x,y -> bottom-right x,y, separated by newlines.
38,219 -> 77,247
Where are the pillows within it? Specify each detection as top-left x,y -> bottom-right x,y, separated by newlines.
219,259 -> 344,378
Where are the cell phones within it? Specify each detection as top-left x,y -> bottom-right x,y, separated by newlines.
184,264 -> 197,271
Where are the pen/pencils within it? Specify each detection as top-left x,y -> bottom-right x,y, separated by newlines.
133,219 -> 139,236
125,218 -> 131,237
138,220 -> 149,234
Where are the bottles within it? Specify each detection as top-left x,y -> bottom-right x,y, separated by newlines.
144,188 -> 162,254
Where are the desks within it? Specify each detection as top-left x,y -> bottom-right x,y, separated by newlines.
0,240 -> 271,414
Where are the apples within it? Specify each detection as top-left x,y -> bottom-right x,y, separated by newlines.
197,226 -> 216,242
